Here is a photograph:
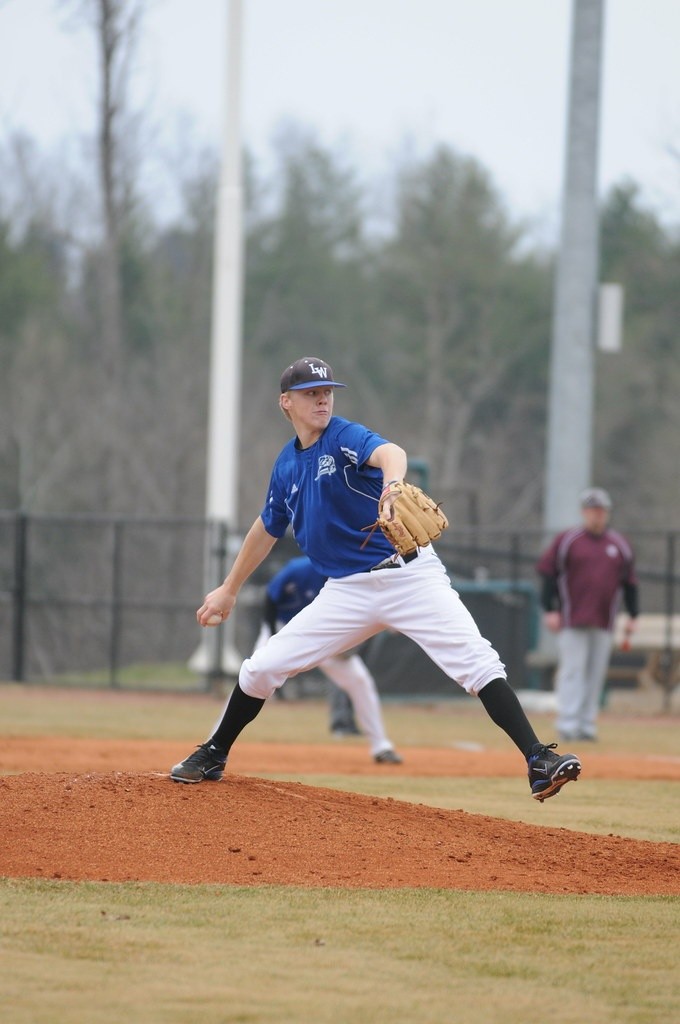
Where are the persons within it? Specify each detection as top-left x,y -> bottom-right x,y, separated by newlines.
170,356 -> 582,803
534,488 -> 641,743
207,554 -> 402,764
327,636 -> 367,737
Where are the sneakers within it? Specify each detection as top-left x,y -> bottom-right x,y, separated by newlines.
169,739 -> 227,783
527,744 -> 581,803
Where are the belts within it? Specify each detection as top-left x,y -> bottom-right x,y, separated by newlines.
372,546 -> 418,572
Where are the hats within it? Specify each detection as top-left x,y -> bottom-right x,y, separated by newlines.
581,488 -> 610,507
281,357 -> 346,391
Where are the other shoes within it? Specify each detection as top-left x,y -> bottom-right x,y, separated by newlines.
376,748 -> 399,765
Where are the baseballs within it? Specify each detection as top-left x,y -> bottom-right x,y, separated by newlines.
207,614 -> 223,625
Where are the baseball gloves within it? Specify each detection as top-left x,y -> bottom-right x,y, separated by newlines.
376,482 -> 450,556
